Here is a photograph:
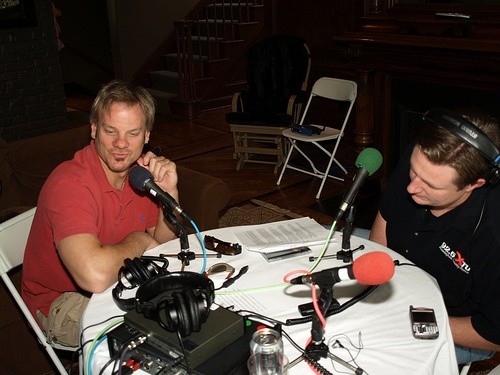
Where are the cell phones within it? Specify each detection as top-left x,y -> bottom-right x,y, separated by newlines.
410,306 -> 440,339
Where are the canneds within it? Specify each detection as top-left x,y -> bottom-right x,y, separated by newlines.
249,328 -> 283,375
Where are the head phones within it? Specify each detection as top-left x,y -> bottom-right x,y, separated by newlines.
421,108 -> 500,186
111,255 -> 215,337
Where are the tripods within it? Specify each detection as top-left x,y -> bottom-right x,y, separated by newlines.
309,206 -> 365,266
158,198 -> 221,271
283,272 -> 363,375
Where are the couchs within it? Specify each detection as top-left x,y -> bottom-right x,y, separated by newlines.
0,126 -> 231,375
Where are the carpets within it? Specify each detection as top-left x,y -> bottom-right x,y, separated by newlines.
218,198 -> 302,229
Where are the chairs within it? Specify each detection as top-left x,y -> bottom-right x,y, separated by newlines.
223,33 -> 313,175
276,76 -> 358,199
0,205 -> 79,375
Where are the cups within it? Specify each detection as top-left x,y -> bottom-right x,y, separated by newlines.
247,350 -> 290,375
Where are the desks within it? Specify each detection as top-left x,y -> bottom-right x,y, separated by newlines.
78,225 -> 459,375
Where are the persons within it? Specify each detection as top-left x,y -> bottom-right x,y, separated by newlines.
19,76 -> 183,348
368,98 -> 500,365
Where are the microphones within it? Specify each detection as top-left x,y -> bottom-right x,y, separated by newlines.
129,166 -> 191,223
335,147 -> 383,221
290,251 -> 396,286
